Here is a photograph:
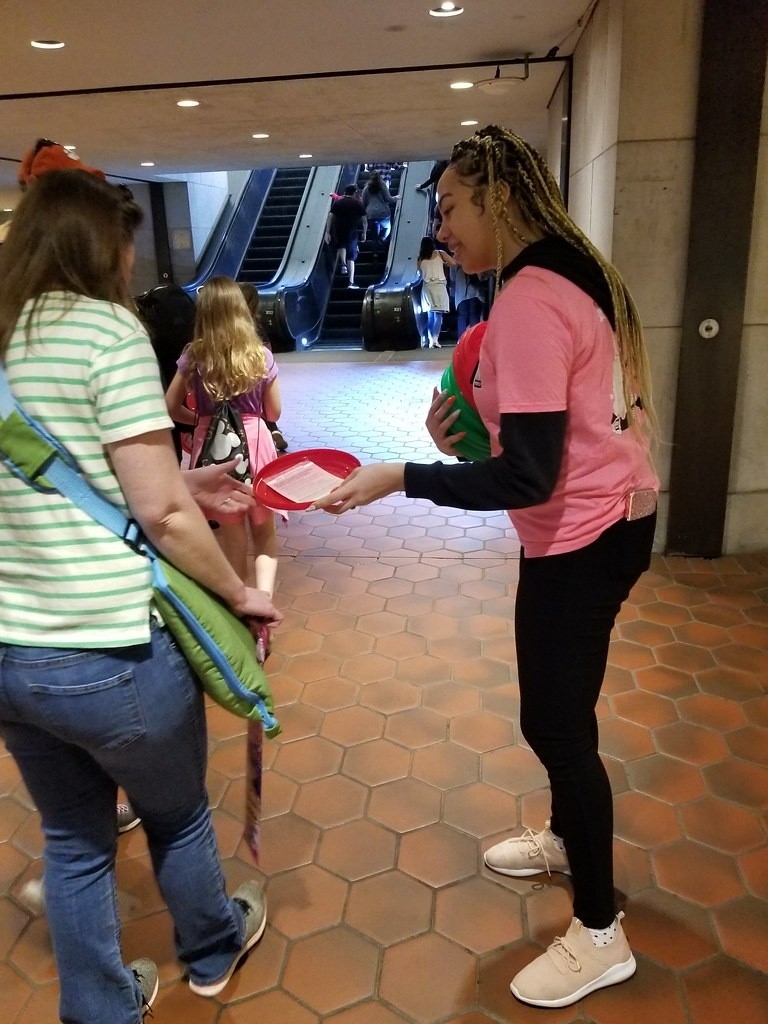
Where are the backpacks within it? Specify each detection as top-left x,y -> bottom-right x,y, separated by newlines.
195,401 -> 254,490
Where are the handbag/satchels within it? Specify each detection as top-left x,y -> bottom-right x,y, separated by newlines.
152,550 -> 283,739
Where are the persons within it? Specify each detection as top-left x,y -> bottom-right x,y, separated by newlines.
306,123 -> 663,1009
164,273 -> 279,669
0,166 -> 280,1024
325,161 -> 485,350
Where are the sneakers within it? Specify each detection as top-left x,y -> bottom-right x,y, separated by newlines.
117,802 -> 141,833
483,819 -> 571,877
188,881 -> 268,997
510,911 -> 637,1006
341,264 -> 348,274
129,956 -> 159,1015
348,282 -> 359,289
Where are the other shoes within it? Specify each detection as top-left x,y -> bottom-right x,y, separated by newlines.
433,342 -> 441,348
272,430 -> 288,450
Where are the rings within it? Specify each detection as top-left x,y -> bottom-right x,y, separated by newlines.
222,497 -> 232,506
